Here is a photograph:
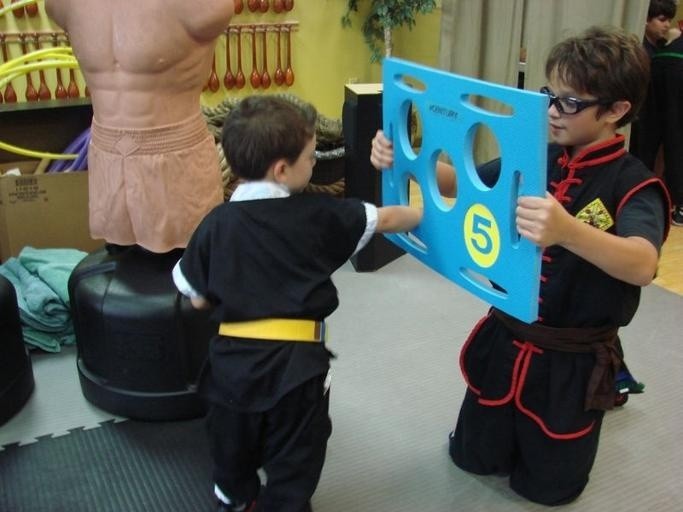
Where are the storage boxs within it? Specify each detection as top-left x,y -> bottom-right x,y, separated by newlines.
0,160 -> 105,267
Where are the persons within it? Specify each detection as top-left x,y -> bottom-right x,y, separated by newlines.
170,92 -> 422,510
368,22 -> 672,506
44,0 -> 235,256
630,0 -> 682,226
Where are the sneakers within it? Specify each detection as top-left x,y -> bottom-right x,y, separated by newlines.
214,484 -> 266,512
613,369 -> 628,406
670,204 -> 683,227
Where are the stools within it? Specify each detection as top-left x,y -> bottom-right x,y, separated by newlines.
0,274 -> 35,432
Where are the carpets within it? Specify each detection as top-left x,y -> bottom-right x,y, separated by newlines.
0,418 -> 234,511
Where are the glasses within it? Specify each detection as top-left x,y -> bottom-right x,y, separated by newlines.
539,86 -> 616,116
